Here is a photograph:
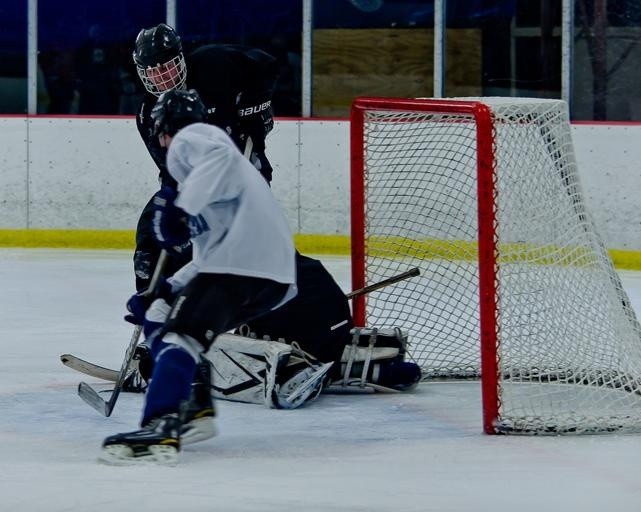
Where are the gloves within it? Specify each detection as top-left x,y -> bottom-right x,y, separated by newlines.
124,279 -> 187,329
151,184 -> 208,253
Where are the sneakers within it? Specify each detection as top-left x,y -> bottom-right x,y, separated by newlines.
102,403 -> 216,458
332,344 -> 422,394
277,364 -> 327,410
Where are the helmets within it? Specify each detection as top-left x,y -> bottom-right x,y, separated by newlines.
132,23 -> 183,70
150,88 -> 205,137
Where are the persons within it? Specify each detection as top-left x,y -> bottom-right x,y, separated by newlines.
190,251 -> 422,412
97,84 -> 298,463
128,19 -> 276,292
